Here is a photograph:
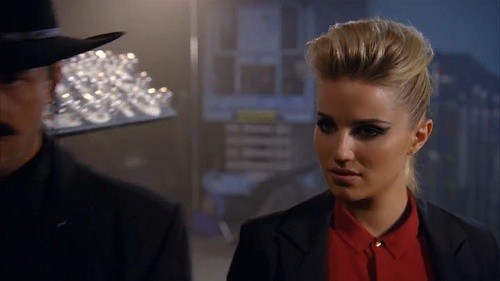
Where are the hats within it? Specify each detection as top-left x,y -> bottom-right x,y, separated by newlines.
0,0 -> 125,83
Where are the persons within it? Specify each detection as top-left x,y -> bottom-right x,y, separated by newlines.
0,0 -> 191,281
225,17 -> 500,281
